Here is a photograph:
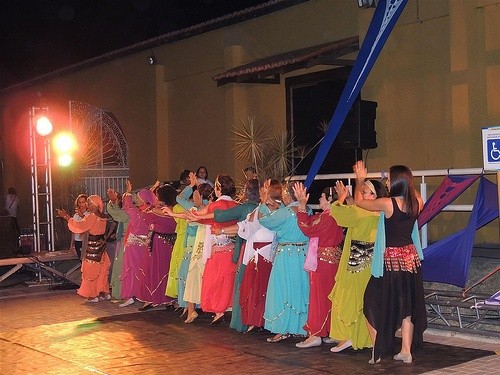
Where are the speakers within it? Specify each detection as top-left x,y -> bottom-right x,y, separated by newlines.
0,216 -> 20,259
341,100 -> 377,149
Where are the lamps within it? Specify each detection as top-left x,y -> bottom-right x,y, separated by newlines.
357,0 -> 377,9
147,56 -> 156,65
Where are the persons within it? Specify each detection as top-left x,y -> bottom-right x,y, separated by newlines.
185,179 -> 261,333
244,167 -> 257,180
354,161 -> 427,364
236,178 -> 283,334
292,181 -> 340,348
4,187 -> 20,230
328,180 -> 388,352
56,166 -> 246,326
257,182 -> 313,343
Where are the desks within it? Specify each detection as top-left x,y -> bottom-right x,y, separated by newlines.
0,253 -> 82,288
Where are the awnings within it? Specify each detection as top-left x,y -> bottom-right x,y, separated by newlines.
211,36 -> 360,88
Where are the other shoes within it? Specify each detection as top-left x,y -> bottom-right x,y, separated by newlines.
369,351 -> 381,364
210,315 -> 226,325
119,298 -> 134,307
293,333 -> 307,338
393,353 -> 412,363
111,299 -> 125,304
295,336 -> 322,348
100,295 -> 111,300
330,339 -> 352,352
85,297 -> 98,302
243,325 -> 261,334
151,303 -> 170,311
169,306 -> 187,318
266,332 -> 290,342
138,302 -> 154,311
323,337 -> 341,344
184,311 -> 199,324
